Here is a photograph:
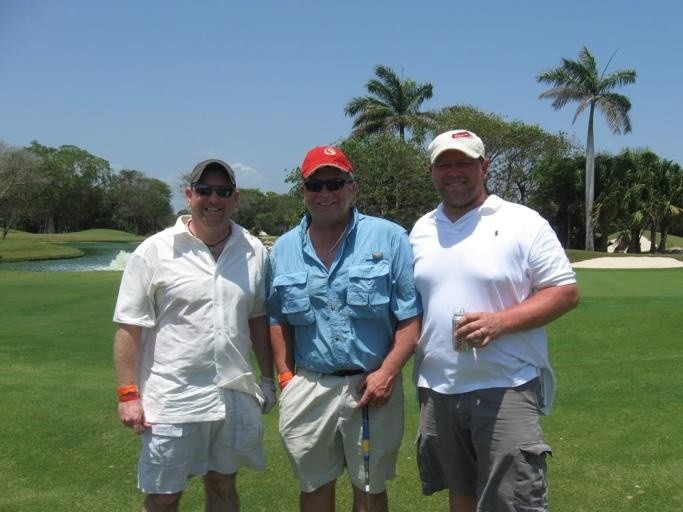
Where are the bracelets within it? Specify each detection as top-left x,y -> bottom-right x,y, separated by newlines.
116,383 -> 138,395
277,380 -> 291,390
277,371 -> 292,383
118,392 -> 139,402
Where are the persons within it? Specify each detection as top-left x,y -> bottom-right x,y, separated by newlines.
262,146 -> 422,511
408,131 -> 580,511
110,159 -> 270,512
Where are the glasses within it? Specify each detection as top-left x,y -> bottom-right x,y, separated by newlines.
305,179 -> 345,192
195,184 -> 233,197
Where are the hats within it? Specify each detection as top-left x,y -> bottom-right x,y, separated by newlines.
430,129 -> 485,164
302,146 -> 352,177
191,159 -> 235,185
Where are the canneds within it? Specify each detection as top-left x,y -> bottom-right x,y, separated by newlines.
452,314 -> 473,353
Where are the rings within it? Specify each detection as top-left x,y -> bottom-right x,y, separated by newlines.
479,328 -> 483,336
